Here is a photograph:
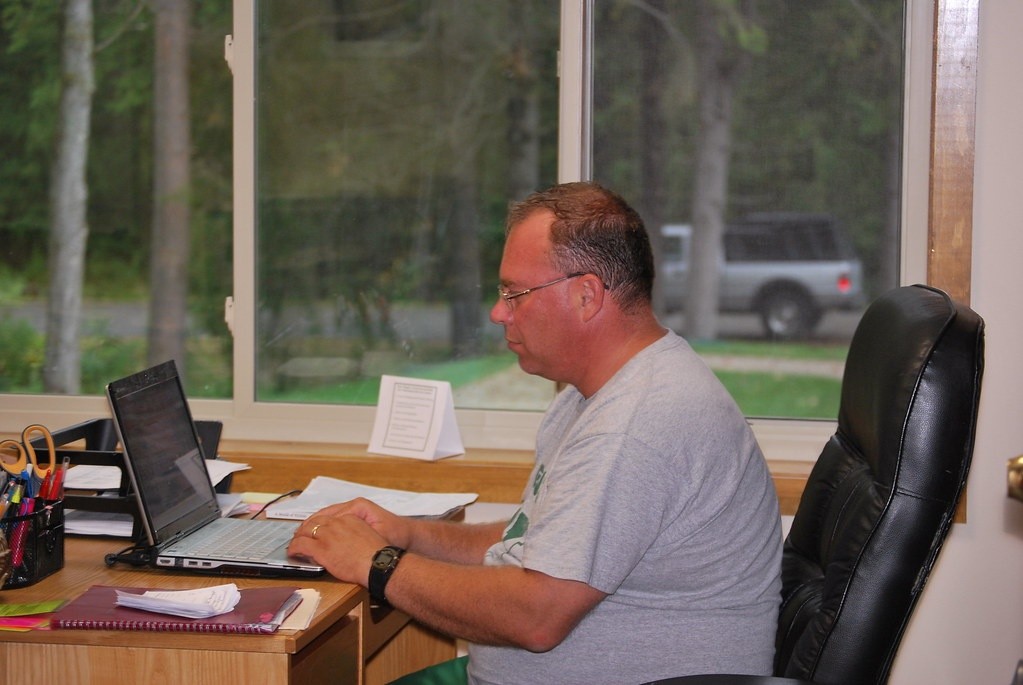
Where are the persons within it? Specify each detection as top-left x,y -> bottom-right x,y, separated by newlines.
287,182 -> 785,685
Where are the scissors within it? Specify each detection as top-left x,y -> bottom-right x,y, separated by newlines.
0,424 -> 55,499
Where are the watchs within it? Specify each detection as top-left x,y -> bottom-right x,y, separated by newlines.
368,546 -> 405,601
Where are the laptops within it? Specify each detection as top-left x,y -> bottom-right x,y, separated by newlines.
105,359 -> 326,577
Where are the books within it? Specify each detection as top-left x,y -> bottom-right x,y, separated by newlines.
50,584 -> 304,634
267,476 -> 479,520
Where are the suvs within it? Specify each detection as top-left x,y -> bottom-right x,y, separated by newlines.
649,209 -> 864,342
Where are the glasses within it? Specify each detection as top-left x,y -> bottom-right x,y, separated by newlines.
497,272 -> 609,310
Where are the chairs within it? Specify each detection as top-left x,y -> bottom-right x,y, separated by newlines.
640,284 -> 986,684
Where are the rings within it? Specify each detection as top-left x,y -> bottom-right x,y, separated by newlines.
312,525 -> 320,538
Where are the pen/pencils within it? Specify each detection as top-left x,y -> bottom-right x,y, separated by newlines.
0,456 -> 71,589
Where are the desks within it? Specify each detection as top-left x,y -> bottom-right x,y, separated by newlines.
0,503 -> 471,685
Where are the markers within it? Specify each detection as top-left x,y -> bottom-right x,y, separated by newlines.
7,498 -> 35,584
